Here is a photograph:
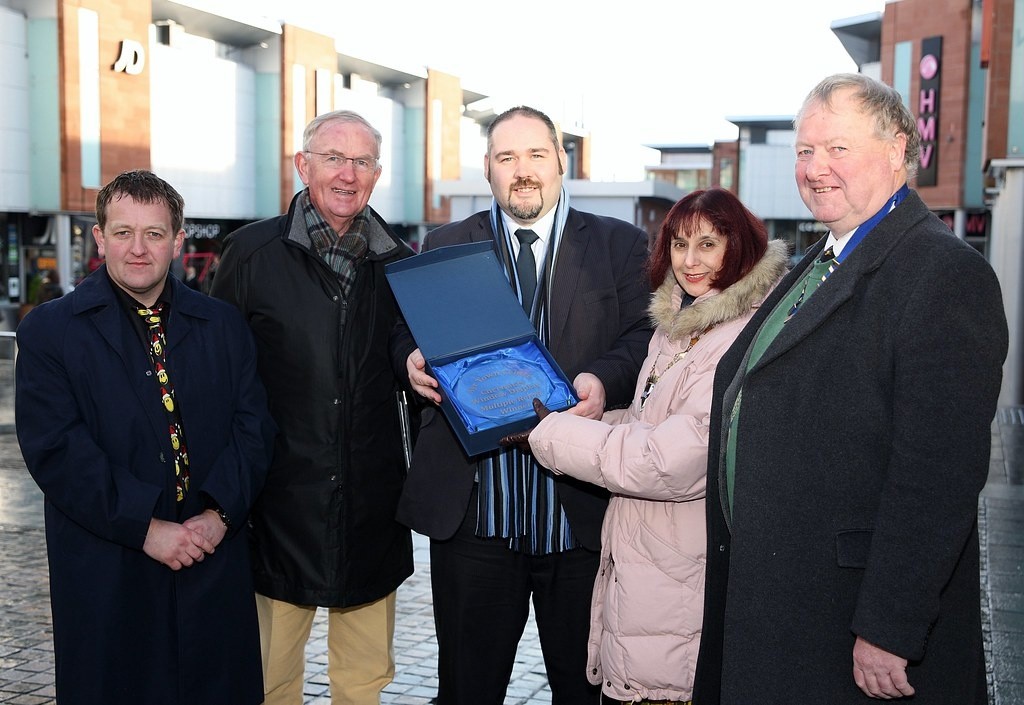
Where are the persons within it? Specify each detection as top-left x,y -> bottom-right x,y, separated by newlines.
391,106 -> 659,705
500,187 -> 787,705
14,170 -> 278,705
209,112 -> 420,705
690,75 -> 1010,705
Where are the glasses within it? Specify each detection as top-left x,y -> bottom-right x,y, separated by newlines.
304,149 -> 380,171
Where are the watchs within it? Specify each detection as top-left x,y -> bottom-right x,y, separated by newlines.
212,503 -> 232,529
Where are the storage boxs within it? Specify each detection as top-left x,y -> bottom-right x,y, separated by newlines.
383,239 -> 582,459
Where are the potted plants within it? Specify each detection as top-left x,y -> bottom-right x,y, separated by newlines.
19,274 -> 43,322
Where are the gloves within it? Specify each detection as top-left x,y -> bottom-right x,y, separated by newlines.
496,398 -> 552,471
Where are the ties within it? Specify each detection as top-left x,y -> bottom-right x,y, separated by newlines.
514,228 -> 540,319
818,245 -> 835,263
135,303 -> 191,503
